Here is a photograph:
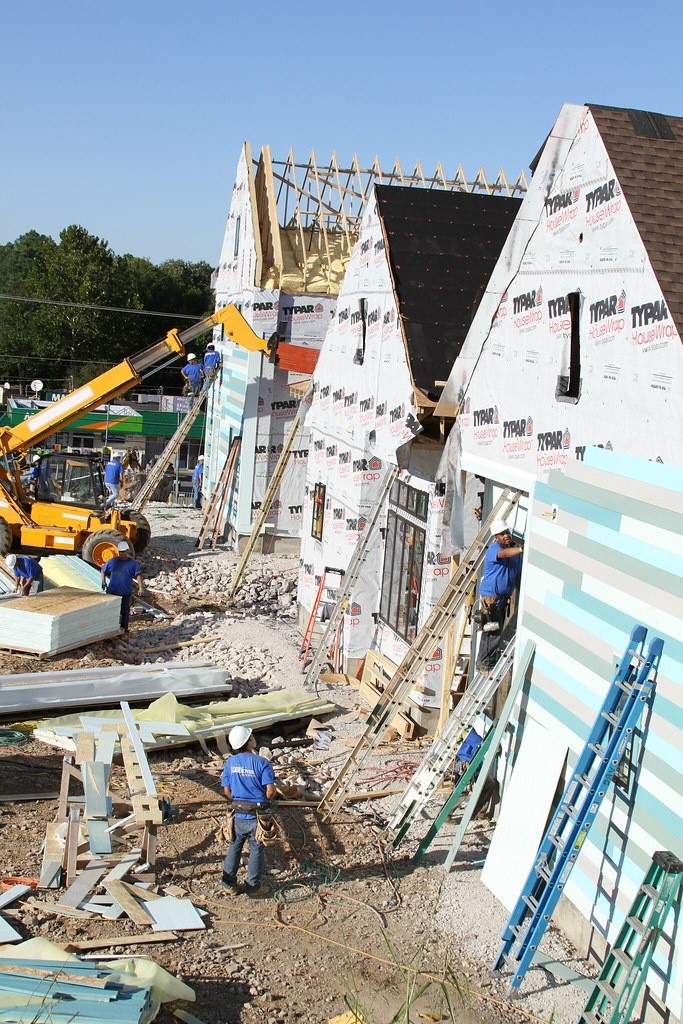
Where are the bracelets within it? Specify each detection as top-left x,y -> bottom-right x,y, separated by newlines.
520,547 -> 523,552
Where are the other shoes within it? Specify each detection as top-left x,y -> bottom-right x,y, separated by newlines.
476,664 -> 481,673
124,629 -> 129,633
480,665 -> 493,674
194,505 -> 202,510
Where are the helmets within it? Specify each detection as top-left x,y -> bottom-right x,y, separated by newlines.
490,519 -> 509,536
187,353 -> 196,361
5,554 -> 17,569
33,455 -> 41,463
117,541 -> 130,552
198,455 -> 204,461
112,452 -> 122,458
206,343 -> 215,351
229,726 -> 252,749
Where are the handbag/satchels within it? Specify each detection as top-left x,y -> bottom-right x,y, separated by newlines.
255,810 -> 280,847
215,809 -> 234,845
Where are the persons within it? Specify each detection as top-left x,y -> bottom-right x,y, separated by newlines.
28,454 -> 55,501
101,453 -> 124,508
476,518 -> 524,676
221,727 -> 276,894
5,554 -> 44,596
180,353 -> 204,394
192,455 -> 204,508
101,541 -> 145,632
200,342 -> 222,398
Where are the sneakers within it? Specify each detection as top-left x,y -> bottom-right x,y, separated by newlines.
222,882 -> 239,896
247,885 -> 269,896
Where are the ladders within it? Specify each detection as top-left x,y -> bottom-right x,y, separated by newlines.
411,717 -> 499,864
316,485 -> 523,826
194,436 -> 242,551
380,624 -> 517,854
303,463 -> 401,694
229,416 -> 300,601
578,851 -> 683,1024
493,624 -> 665,996
298,565 -> 346,673
129,362 -> 221,513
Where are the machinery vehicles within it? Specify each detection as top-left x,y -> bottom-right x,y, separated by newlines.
0,300 -> 279,574
